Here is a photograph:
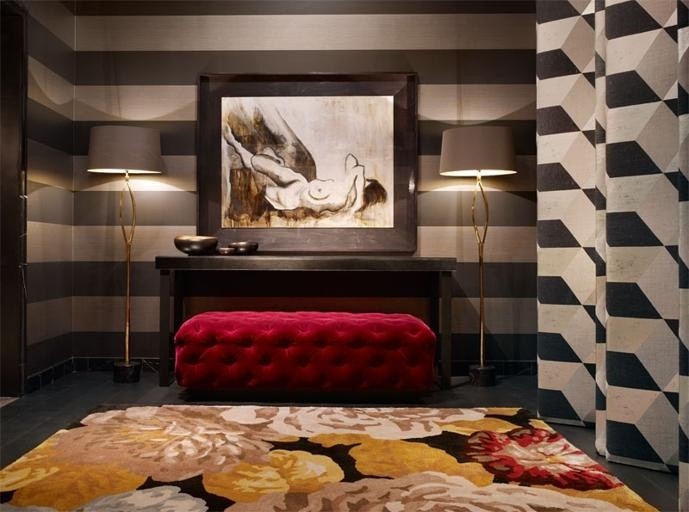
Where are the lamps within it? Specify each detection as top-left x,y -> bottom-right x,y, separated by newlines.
86,125 -> 165,383
439,126 -> 519,388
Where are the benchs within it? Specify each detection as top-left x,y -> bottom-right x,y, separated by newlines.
174,310 -> 438,403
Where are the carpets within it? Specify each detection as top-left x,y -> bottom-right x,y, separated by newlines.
1,396 -> 664,512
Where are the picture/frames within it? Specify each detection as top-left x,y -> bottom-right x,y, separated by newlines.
196,71 -> 420,251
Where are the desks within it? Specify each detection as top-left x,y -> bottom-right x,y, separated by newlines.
153,255 -> 457,390
